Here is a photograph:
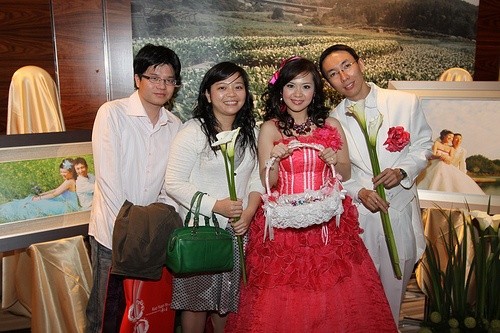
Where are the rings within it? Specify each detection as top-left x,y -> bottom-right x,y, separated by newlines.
375,201 -> 378,204
331,156 -> 334,160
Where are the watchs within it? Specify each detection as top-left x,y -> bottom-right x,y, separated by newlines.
399,169 -> 407,180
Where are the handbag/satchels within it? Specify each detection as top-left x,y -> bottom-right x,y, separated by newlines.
165,191 -> 234,277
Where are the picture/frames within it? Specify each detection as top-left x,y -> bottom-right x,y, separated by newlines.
387,81 -> 500,215
1,130 -> 95,251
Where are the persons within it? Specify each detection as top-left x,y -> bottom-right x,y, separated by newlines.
82,42 -> 184,333
319,44 -> 433,328
0,158 -> 81,222
163,61 -> 266,333
223,56 -> 399,333
415,129 -> 486,195
72,157 -> 95,209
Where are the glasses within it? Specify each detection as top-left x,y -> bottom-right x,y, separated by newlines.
326,60 -> 357,82
140,74 -> 177,87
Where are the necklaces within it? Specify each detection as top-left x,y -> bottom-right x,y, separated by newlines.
290,118 -> 315,136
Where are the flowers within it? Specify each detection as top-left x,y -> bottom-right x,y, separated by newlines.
383,125 -> 411,153
211,127 -> 246,286
416,197 -> 500,333
345,99 -> 403,278
